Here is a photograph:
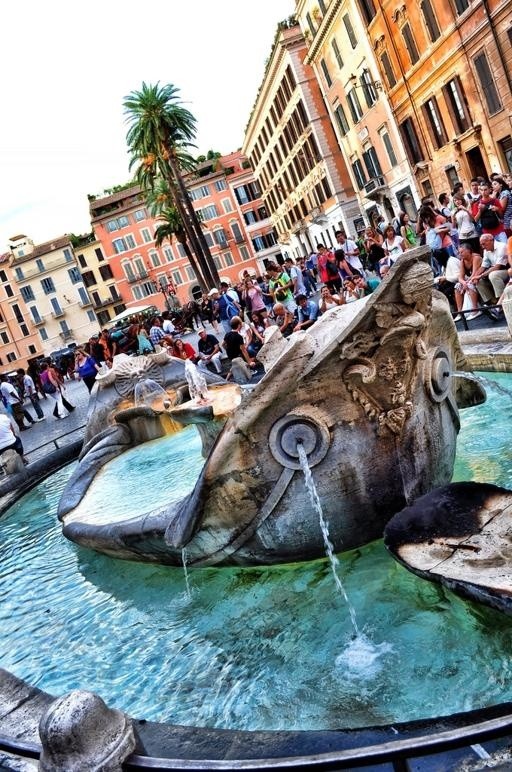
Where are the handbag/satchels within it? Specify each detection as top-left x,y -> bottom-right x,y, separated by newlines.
245,289 -> 253,311
42,382 -> 56,394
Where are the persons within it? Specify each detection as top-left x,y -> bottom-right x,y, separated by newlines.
165,174 -> 512,386
0,305 -> 165,460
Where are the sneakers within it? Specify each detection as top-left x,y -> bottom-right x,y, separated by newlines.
467,310 -> 483,321
22,405 -> 77,432
453,313 -> 462,323
480,304 -> 500,319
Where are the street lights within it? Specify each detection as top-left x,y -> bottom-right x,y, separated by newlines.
151,275 -> 173,310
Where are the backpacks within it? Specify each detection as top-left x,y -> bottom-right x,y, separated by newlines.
326,260 -> 339,277
476,200 -> 500,229
223,294 -> 238,318
272,280 -> 288,302
424,229 -> 443,248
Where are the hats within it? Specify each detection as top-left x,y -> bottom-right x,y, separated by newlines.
207,287 -> 219,297
197,325 -> 205,335
